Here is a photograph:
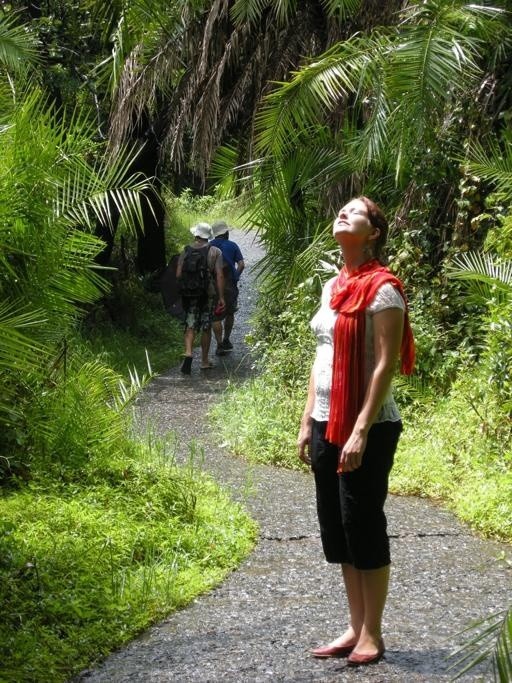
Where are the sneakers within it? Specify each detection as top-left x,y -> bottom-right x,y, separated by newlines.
216,339 -> 233,355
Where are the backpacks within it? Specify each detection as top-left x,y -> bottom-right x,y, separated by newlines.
180,244 -> 213,299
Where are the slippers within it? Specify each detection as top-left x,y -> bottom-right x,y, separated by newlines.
181,357 -> 192,374
200,362 -> 216,369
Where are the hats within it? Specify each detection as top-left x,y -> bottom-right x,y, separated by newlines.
190,222 -> 212,239
212,221 -> 233,238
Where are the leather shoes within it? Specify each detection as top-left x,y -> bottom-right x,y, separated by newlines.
311,638 -> 385,665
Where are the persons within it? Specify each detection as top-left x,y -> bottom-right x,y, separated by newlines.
208,220 -> 246,353
295,195 -> 410,664
173,220 -> 226,374
